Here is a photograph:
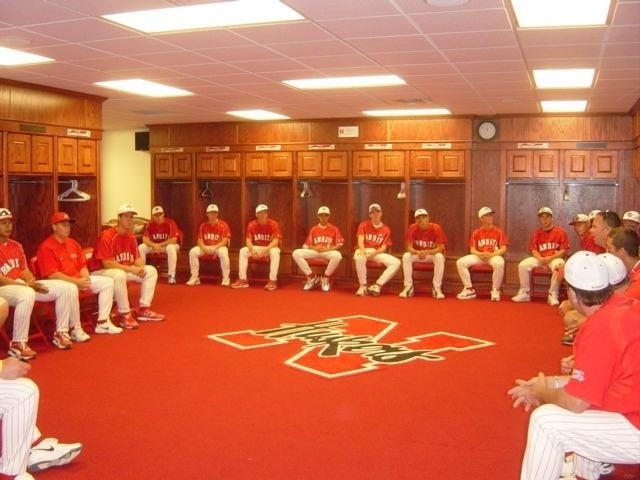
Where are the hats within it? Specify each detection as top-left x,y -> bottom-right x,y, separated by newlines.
369,204 -> 382,213
597,251 -> 627,285
537,206 -> 553,214
415,209 -> 428,217
50,213 -> 75,223
588,211 -> 599,221
206,204 -> 218,212
255,204 -> 269,214
623,211 -> 640,220
478,206 -> 496,217
565,250 -> 608,290
569,214 -> 586,226
118,204 -> 139,216
318,207 -> 331,216
151,205 -> 164,214
0,208 -> 18,222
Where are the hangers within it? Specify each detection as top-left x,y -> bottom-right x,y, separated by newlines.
57,180 -> 90,202
397,182 -> 406,200
201,182 -> 214,197
300,182 -> 313,199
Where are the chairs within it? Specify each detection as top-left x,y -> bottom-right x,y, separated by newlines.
0,306 -> 53,354
304,258 -> 338,293
198,252 -> 224,284
30,256 -> 86,338
468,264 -> 504,299
147,228 -> 184,282
246,233 -> 283,290
530,254 -> 567,301
412,262 -> 447,298
80,247 -> 138,330
352,237 -> 393,295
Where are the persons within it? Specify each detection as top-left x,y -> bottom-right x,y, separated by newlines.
456,206 -> 509,302
508,250 -> 640,480
137,206 -> 182,285
185,205 -> 231,286
588,210 -> 602,227
0,207 -> 73,360
568,213 -> 606,254
0,298 -> 82,480
605,228 -> 640,284
353,203 -> 401,296
231,204 -> 282,291
292,205 -> 344,293
560,253 -> 640,374
89,205 -> 166,330
561,209 -> 623,345
36,211 -> 123,342
399,208 -> 446,299
621,210 -> 640,235
512,207 -> 570,306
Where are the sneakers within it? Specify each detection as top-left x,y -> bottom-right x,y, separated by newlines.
70,326 -> 91,343
221,277 -> 230,286
432,287 -> 445,300
355,285 -> 369,296
232,279 -> 251,289
137,306 -> 166,322
1,469 -> 33,480
491,288 -> 502,303
369,283 -> 384,296
52,333 -> 73,349
168,275 -> 176,285
512,289 -> 531,303
186,276 -> 201,286
264,279 -> 276,290
28,439 -> 84,471
95,319 -> 122,336
399,285 -> 416,298
321,275 -> 330,294
561,457 -> 614,476
121,313 -> 139,329
546,293 -> 560,306
302,275 -> 318,292
9,348 -> 37,360
456,287 -> 478,301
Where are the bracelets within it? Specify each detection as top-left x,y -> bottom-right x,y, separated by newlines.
554,375 -> 559,389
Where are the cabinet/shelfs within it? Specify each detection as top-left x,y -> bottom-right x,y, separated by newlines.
193,152 -> 244,275
150,152 -> 193,274
407,150 -> 473,293
244,152 -> 295,279
52,126 -> 103,251
505,148 -> 560,287
295,151 -> 350,286
349,150 -> 407,294
1,120 -> 53,251
563,149 -> 618,258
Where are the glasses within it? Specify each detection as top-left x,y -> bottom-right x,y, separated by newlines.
605,208 -> 610,217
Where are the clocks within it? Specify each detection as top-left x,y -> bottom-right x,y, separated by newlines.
478,121 -> 496,140
